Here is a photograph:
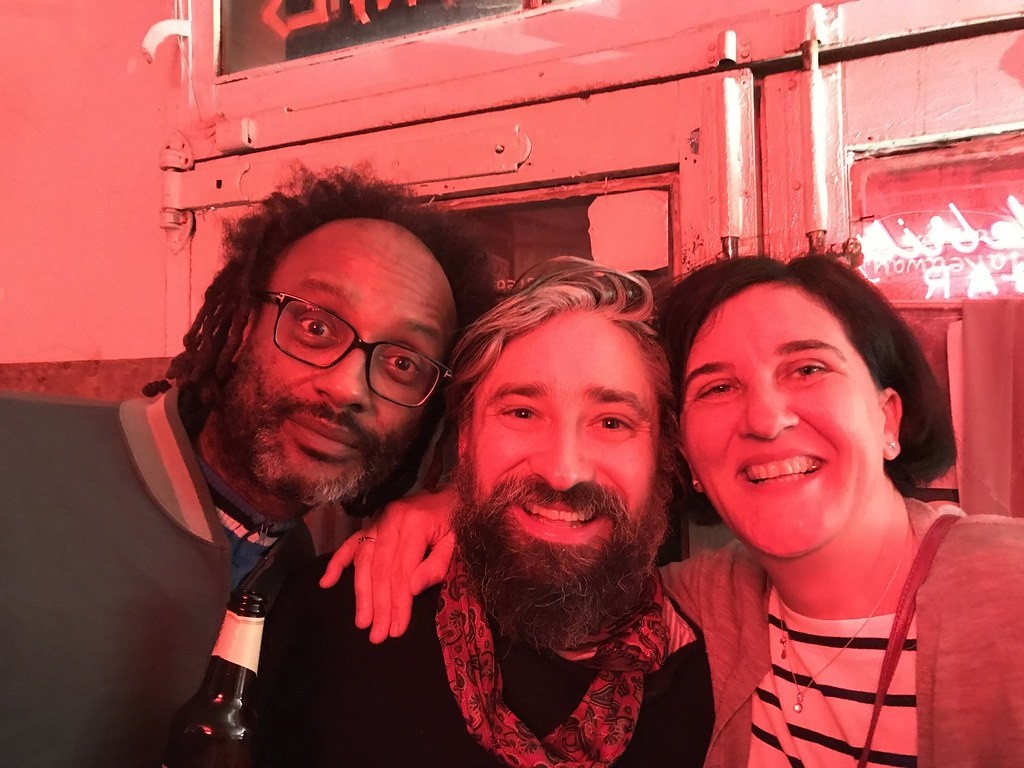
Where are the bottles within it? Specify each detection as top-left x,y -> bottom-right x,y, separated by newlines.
161,588 -> 270,768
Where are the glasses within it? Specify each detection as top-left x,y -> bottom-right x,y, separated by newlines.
255,292 -> 454,410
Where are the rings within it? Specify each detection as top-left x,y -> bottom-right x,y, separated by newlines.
358,537 -> 375,543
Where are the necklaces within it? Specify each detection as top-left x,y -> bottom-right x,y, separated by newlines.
779,530 -> 910,713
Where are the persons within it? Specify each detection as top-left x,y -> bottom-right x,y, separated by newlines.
323,254 -> 1023,768
239,253 -> 714,768
0,169 -> 494,767
